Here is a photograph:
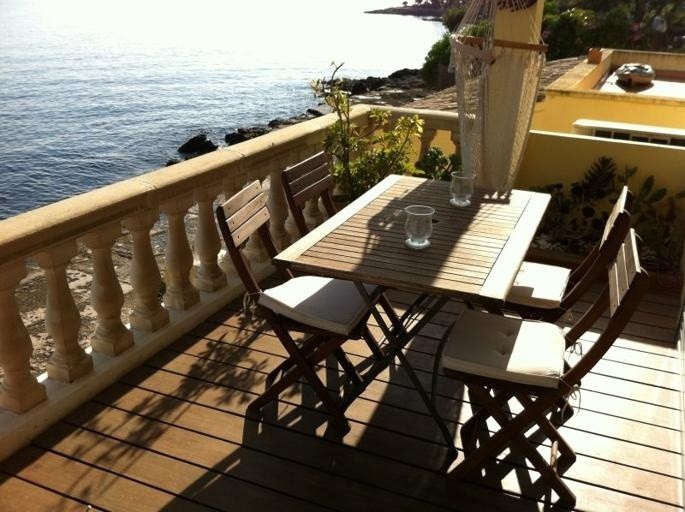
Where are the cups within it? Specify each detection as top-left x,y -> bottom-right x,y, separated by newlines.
405,205 -> 436,250
450,170 -> 475,208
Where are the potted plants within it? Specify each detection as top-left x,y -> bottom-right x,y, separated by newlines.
309,59 -> 685,291
